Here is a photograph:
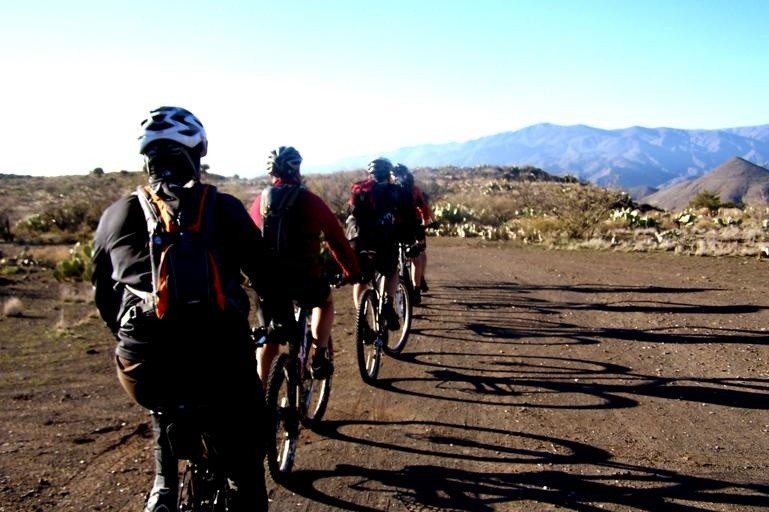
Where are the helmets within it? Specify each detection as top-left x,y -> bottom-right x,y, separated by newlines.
368,158 -> 391,177
138,106 -> 207,157
391,162 -> 408,180
266,147 -> 301,177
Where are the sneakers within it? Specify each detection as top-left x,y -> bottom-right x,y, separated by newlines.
413,286 -> 421,306
311,357 -> 334,379
420,281 -> 428,292
144,487 -> 176,512
363,315 -> 375,344
383,298 -> 400,330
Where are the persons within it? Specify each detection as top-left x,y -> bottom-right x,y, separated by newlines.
246,146 -> 361,435
345,158 -> 427,345
389,162 -> 440,309
88,105 -> 296,511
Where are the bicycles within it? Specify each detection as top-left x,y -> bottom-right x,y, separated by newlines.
148,325 -> 297,510
254,254 -> 372,483
346,221 -> 440,386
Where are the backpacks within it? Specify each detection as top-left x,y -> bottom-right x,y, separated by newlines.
258,183 -> 308,266
127,180 -> 234,325
391,184 -> 416,228
346,179 -> 387,242
